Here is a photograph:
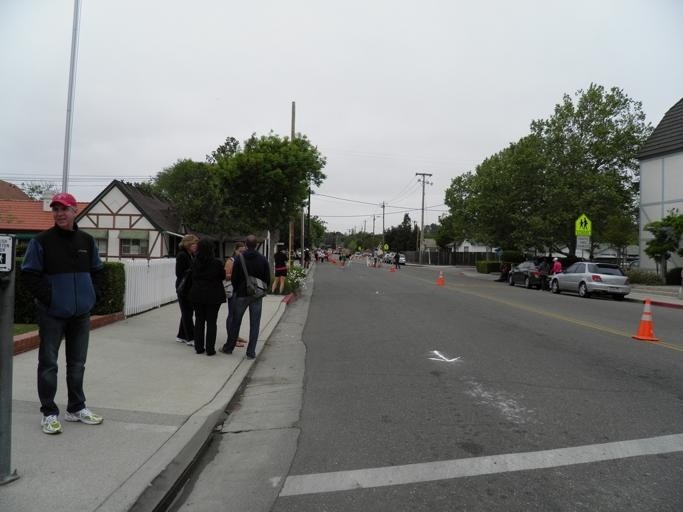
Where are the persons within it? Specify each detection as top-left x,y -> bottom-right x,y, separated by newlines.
176,234 -> 270,359
271,247 -> 288,293
20,194 -> 105,434
500,257 -> 561,290
304,248 -> 400,269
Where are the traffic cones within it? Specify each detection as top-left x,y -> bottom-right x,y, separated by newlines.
628,299 -> 659,342
436,271 -> 444,287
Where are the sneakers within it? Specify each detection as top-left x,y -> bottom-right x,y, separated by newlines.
219,345 -> 225,352
235,337 -> 247,347
176,337 -> 194,346
41,414 -> 63,434
64,408 -> 103,425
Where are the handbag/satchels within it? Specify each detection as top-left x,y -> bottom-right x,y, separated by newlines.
222,279 -> 233,298
176,272 -> 193,297
247,276 -> 268,299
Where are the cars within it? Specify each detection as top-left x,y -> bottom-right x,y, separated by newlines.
505,259 -> 630,301
351,249 -> 407,265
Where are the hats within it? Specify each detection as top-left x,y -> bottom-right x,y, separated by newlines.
50,193 -> 78,207
